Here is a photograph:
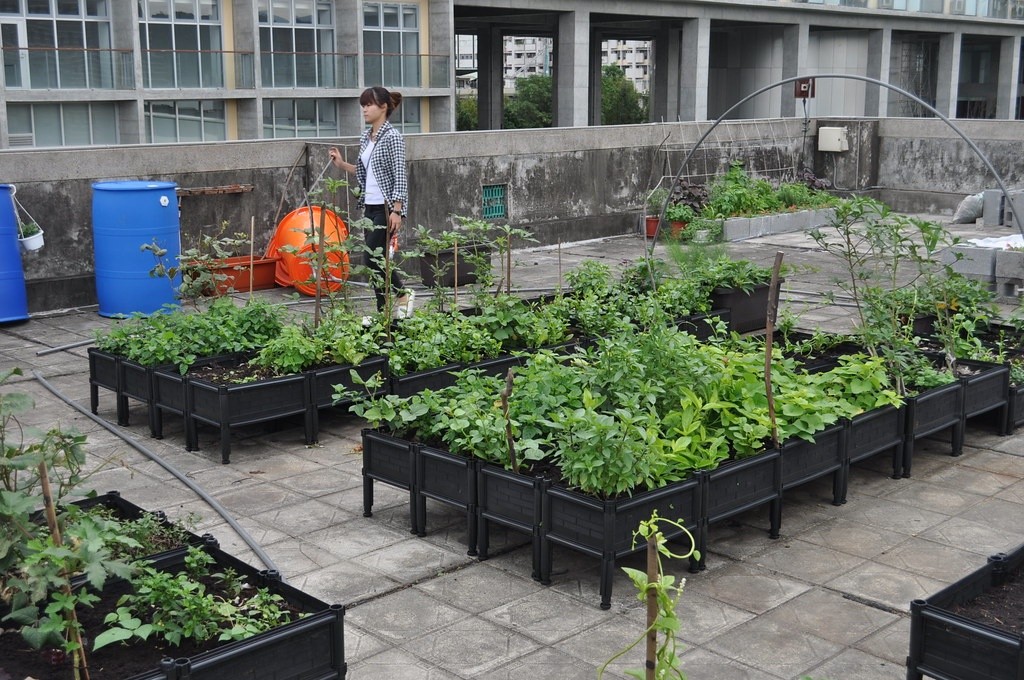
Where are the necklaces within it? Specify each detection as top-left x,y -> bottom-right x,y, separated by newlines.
371,135 -> 375,138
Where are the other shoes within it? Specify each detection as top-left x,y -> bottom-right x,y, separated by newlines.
373,312 -> 384,322
398,288 -> 416,319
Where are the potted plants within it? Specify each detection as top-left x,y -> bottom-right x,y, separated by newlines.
0,156 -> 1024,679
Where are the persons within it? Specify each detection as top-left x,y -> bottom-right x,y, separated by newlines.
329,87 -> 415,326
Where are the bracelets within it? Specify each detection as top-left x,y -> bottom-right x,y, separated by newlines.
394,210 -> 401,216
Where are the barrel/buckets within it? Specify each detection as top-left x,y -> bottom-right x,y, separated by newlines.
92,180 -> 182,318
267,205 -> 350,296
0,183 -> 30,323
